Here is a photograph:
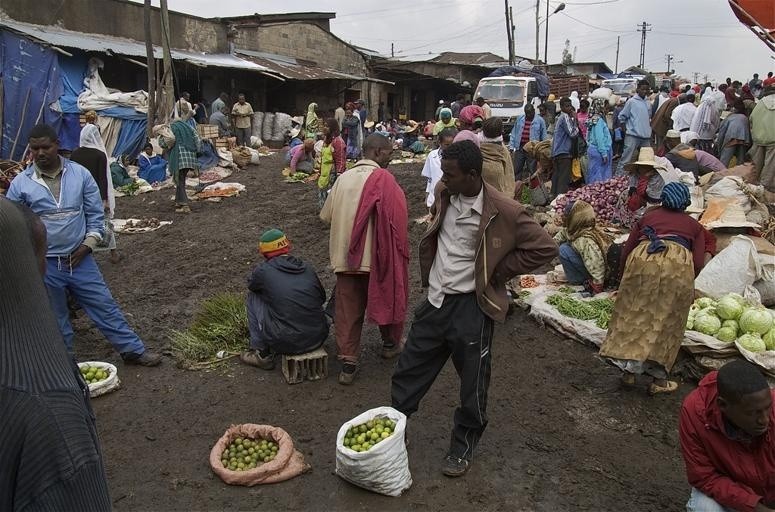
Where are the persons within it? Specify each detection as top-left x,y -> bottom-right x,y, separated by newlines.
239,228 -> 332,370
319,132 -> 411,385
389,139 -> 561,478
80,90 -> 255,214
5,123 -> 163,366
598,181 -> 705,395
284,65 -> 775,309
1,196 -> 111,512
678,362 -> 775,512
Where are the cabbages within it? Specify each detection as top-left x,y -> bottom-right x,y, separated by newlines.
686,293 -> 775,352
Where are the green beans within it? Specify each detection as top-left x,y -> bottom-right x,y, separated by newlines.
544,293 -> 615,330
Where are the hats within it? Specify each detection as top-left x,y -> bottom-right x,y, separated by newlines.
624,147 -> 666,170
258,229 -> 289,259
666,130 -> 680,138
354,99 -> 364,104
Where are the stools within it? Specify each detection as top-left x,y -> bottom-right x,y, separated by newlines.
280,346 -> 329,385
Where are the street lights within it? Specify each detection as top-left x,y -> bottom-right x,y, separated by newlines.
536,2 -> 565,66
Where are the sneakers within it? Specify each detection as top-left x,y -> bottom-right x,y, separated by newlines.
241,350 -> 274,369
443,455 -> 471,476
338,364 -> 357,385
622,372 -> 679,396
122,348 -> 161,366
381,344 -> 399,358
175,206 -> 190,214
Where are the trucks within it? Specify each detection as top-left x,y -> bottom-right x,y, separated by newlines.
472,75 -> 589,140
600,72 -> 673,97
